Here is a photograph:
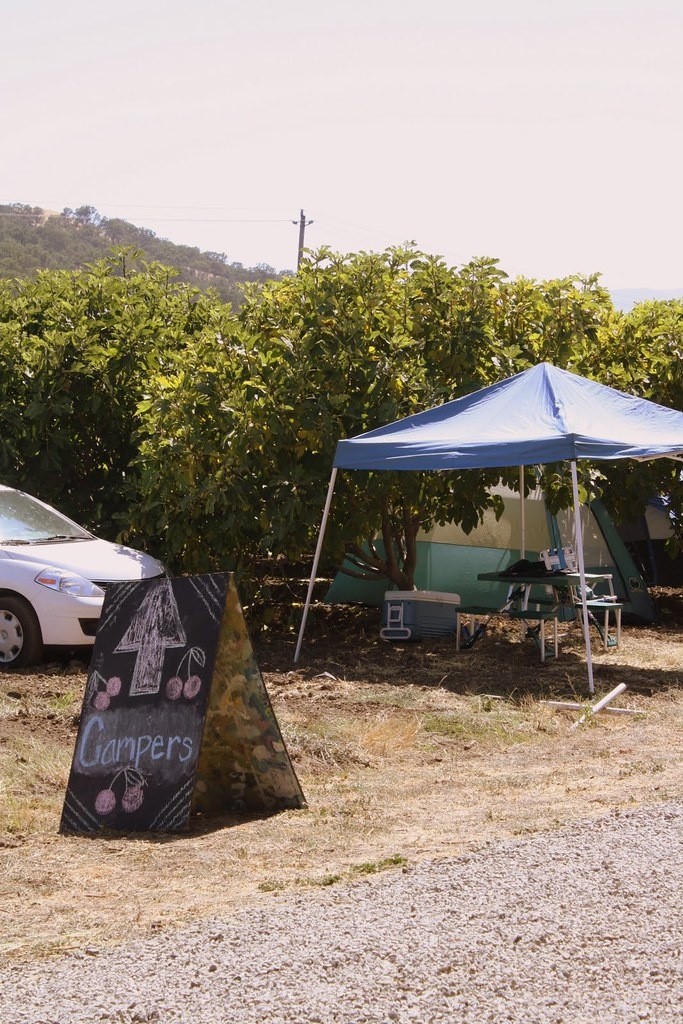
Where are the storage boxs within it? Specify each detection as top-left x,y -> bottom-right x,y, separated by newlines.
377,590 -> 461,645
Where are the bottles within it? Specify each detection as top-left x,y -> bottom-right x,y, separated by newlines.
467,619 -> 474,637
461,623 -> 469,641
475,618 -> 480,633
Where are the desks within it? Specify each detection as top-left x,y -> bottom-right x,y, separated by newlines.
478,570 -> 617,658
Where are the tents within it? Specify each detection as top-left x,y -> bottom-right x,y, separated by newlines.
294,364 -> 682,693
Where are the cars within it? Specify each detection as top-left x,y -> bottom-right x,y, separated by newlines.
0,485 -> 169,671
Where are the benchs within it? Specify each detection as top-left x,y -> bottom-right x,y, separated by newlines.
454,601 -> 559,664
579,600 -> 623,651
